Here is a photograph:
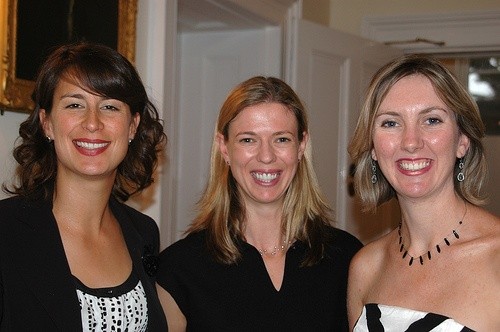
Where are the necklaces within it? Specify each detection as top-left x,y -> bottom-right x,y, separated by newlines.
259,238 -> 291,256
397,202 -> 468,266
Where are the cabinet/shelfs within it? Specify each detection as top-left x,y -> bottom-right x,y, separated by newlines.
0,0 -> 138,114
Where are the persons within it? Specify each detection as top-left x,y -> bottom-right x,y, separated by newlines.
0,44 -> 167,332
154,76 -> 364,332
345,56 -> 500,332
